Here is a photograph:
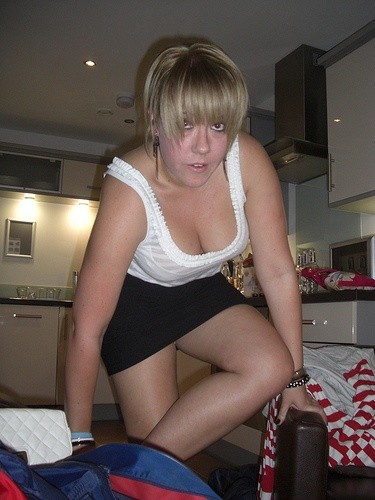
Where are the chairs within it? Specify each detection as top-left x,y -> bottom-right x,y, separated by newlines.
256,343 -> 375,500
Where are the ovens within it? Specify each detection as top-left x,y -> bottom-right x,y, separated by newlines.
211,306 -> 273,434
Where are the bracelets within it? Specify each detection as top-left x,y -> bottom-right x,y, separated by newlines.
290,367 -> 308,380
69,432 -> 96,448
288,376 -> 312,389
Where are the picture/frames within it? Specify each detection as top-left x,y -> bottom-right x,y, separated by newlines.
329,234 -> 375,279
5,218 -> 36,258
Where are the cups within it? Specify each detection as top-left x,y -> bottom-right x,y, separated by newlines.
17,287 -> 62,299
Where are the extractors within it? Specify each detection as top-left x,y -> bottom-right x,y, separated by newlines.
260,44 -> 329,186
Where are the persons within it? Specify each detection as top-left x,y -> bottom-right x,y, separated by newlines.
62,41 -> 328,464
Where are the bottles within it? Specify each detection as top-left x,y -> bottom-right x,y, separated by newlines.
295,247 -> 317,295
222,260 -> 244,295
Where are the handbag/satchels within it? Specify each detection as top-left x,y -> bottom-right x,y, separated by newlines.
299,266 -> 375,290
0,442 -> 222,500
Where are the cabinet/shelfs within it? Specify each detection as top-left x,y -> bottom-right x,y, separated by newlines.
0,303 -> 123,422
176,306 -> 267,457
316,20 -> 375,215
302,300 -> 375,351
0,141 -> 114,202
